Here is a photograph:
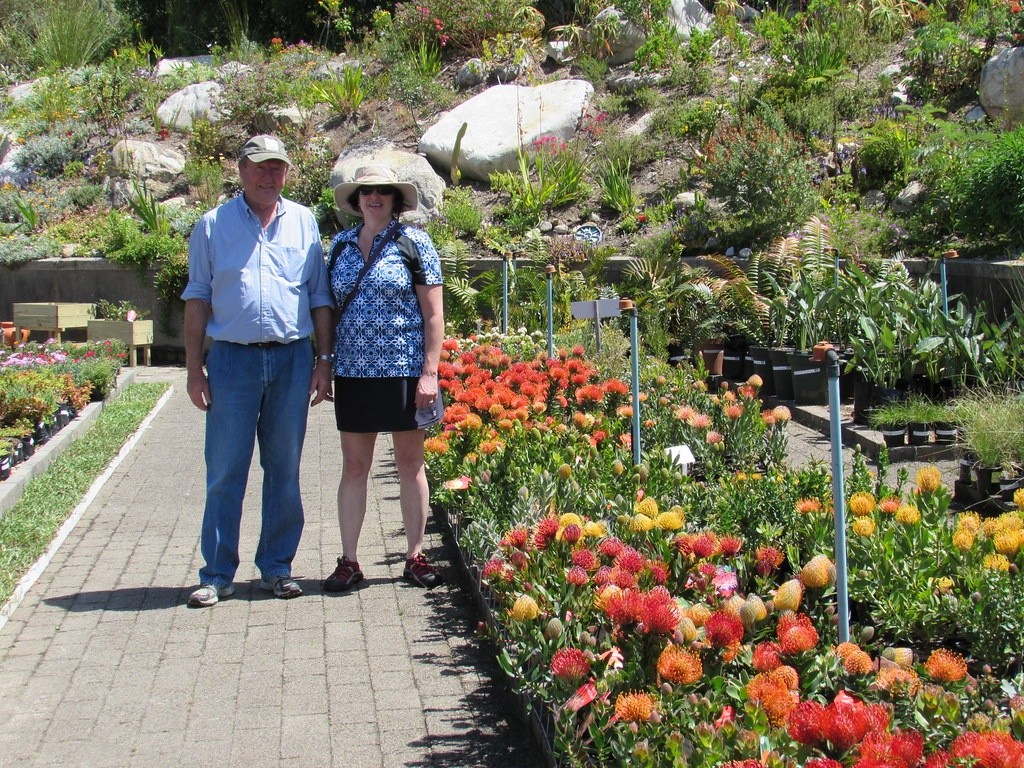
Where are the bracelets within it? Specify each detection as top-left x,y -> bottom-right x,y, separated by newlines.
317,354 -> 332,363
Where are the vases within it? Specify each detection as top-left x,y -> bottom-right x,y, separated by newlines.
13,302 -> 97,343
88,319 -> 154,367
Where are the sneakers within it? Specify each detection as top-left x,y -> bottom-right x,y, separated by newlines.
327,556 -> 363,590
403,552 -> 442,587
260,573 -> 303,598
188,582 -> 234,606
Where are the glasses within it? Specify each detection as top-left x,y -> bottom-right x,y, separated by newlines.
356,185 -> 396,195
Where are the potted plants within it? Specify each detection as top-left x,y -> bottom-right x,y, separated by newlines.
628,215 -> 974,447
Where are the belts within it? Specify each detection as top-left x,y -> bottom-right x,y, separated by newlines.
226,341 -> 286,348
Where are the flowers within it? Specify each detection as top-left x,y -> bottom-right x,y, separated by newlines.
91,298 -> 150,322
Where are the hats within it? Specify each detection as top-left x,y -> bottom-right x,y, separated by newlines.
239,134 -> 291,165
334,165 -> 418,217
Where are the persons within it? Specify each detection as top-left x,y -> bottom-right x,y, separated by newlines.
323,160 -> 446,592
179,135 -> 334,607
